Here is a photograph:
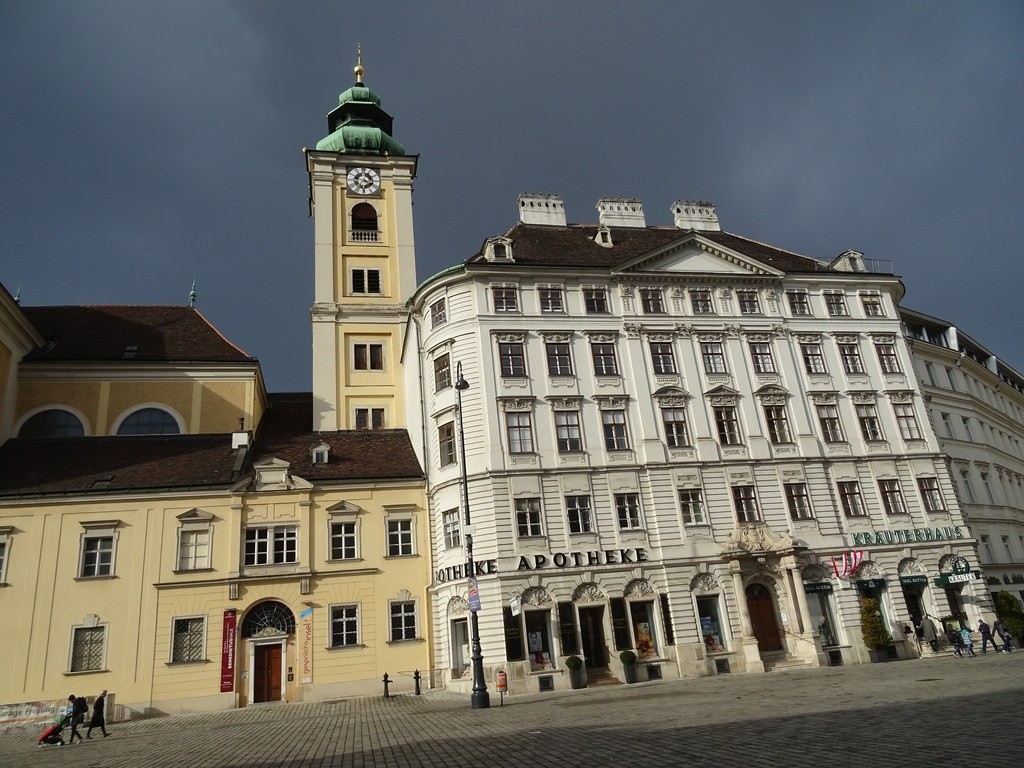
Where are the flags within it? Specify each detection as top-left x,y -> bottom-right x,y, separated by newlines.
831,550 -> 864,577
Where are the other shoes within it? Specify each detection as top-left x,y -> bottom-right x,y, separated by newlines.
76,738 -> 84,745
1002,650 -> 1006,655
86,736 -> 93,739
65,741 -> 72,745
103,733 -> 111,738
960,656 -> 964,658
972,654 -> 977,657
968,655 -> 972,657
952,654 -> 958,658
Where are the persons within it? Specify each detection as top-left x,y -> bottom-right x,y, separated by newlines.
65,694 -> 85,745
86,689 -> 112,739
915,614 -> 942,652
946,616 -> 1013,658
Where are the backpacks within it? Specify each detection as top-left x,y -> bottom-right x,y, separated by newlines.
947,631 -> 955,643
81,697 -> 89,713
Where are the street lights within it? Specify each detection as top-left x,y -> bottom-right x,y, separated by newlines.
454,360 -> 490,709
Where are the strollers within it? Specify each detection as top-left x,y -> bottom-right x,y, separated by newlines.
38,711 -> 73,748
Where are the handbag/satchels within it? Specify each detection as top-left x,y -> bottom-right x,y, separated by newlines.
1003,632 -> 1012,640
